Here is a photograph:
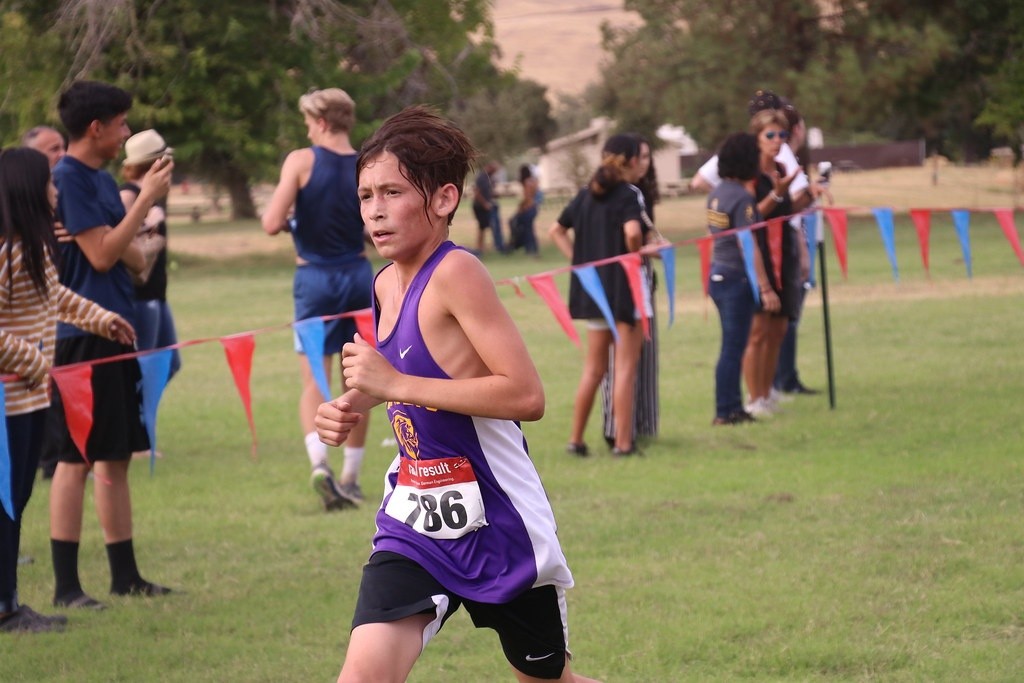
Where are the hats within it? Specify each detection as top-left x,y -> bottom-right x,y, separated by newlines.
123,130 -> 173,166
604,134 -> 643,161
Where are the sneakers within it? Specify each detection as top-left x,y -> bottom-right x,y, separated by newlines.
744,388 -> 785,420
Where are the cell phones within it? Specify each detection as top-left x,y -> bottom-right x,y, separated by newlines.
155,154 -> 172,173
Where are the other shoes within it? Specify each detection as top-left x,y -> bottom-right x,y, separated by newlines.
0,601 -> 68,632
53,588 -> 107,611
311,464 -> 365,511
714,413 -> 756,425
567,444 -> 645,458
775,382 -> 818,394
111,580 -> 172,597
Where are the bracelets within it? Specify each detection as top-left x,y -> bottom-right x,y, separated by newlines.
758,283 -> 774,294
804,187 -> 812,197
770,191 -> 784,203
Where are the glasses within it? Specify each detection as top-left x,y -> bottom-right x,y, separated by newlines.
766,130 -> 787,139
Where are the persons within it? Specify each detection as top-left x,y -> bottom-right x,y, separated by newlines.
259,85 -> 369,513
312,102 -> 604,683
550,133 -> 675,457
19,125 -> 183,479
0,146 -> 138,634
49,79 -> 175,612
690,88 -> 829,426
471,162 -> 546,257
